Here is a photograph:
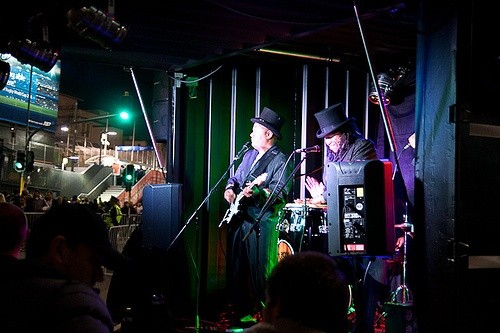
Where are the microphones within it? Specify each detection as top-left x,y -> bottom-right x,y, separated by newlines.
237,141 -> 251,157
295,145 -> 321,154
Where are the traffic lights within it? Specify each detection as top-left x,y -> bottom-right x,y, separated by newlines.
119,95 -> 134,124
126,165 -> 134,182
14,149 -> 26,172
27,151 -> 35,172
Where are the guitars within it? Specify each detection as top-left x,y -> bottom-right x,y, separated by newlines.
217,171 -> 268,228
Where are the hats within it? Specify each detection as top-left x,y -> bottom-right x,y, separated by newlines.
30,204 -> 133,272
314,103 -> 355,138
250,107 -> 286,140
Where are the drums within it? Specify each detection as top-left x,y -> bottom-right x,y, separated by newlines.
278,202 -> 328,262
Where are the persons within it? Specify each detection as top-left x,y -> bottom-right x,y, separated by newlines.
0,189 -> 143,239
246,250 -> 351,333
0,202 -> 114,333
305,103 -> 377,204
223,107 -> 292,295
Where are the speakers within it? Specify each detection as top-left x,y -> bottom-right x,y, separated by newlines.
142,182 -> 185,333
326,158 -> 384,257
